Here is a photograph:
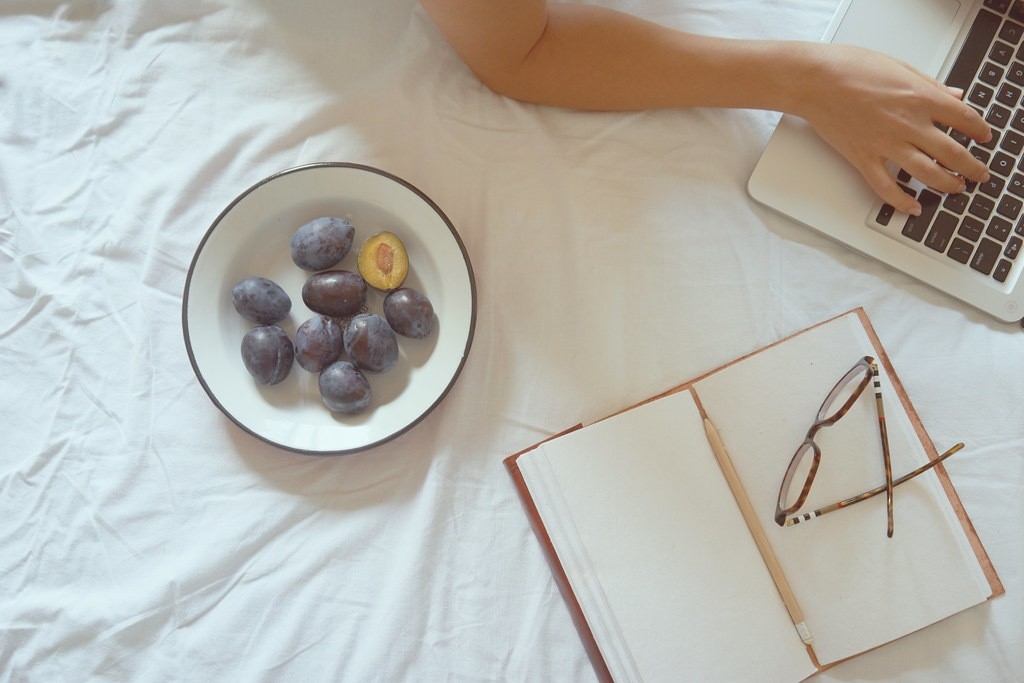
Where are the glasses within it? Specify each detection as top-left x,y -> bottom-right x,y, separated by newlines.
775,356 -> 965,539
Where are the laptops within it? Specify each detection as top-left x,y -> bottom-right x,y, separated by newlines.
745,0 -> 1024,322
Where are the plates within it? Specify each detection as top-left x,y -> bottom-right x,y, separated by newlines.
183,161 -> 478,455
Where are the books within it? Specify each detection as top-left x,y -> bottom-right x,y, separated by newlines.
501,306 -> 1005,683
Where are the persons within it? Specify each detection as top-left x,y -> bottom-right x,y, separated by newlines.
423,0 -> 993,219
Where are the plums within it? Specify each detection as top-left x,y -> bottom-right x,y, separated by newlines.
231,216 -> 434,413
